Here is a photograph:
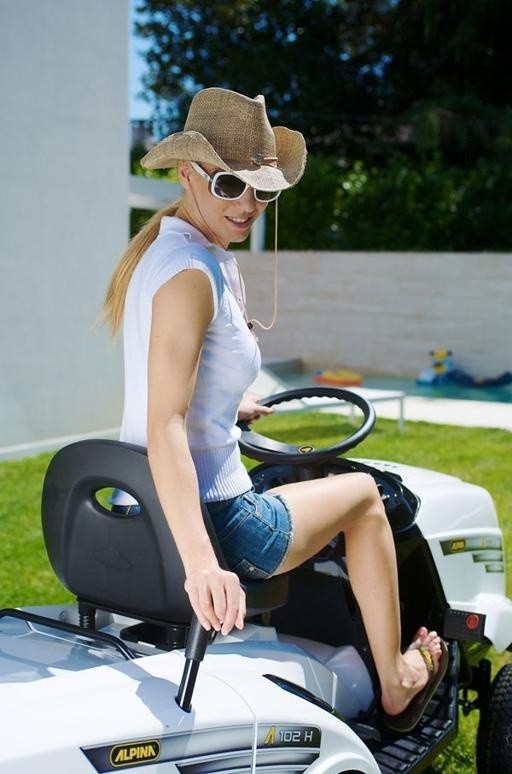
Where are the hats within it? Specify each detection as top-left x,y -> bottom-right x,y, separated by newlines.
140,87 -> 307,194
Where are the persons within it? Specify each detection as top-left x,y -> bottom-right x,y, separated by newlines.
100,87 -> 450,735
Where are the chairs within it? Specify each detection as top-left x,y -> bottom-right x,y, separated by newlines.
250,365 -> 404,429
39,439 -> 292,713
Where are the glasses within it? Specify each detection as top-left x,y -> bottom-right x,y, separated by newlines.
192,156 -> 284,205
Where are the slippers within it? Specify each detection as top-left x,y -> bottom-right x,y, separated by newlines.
383,632 -> 449,730
